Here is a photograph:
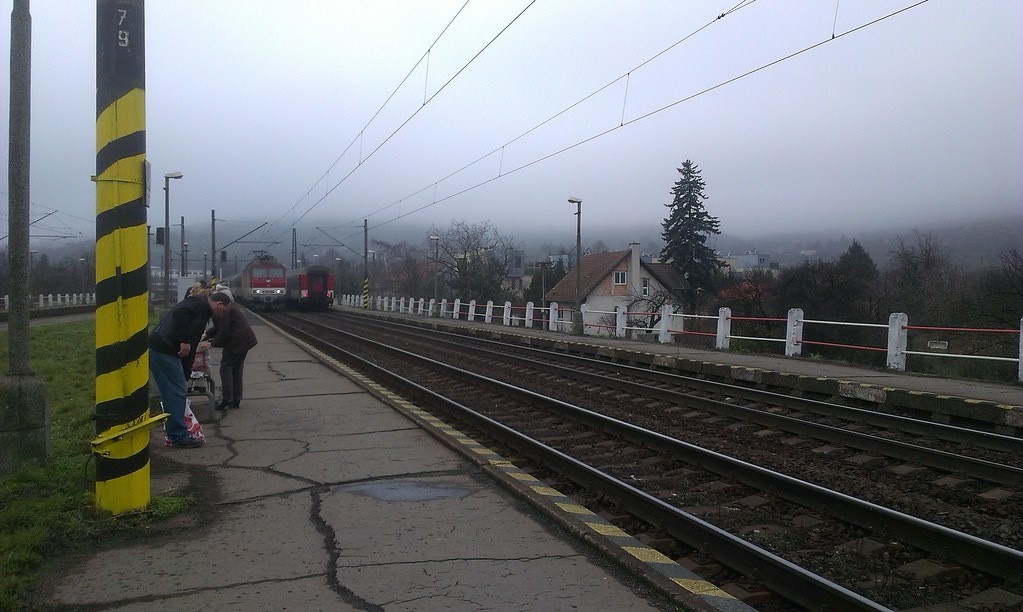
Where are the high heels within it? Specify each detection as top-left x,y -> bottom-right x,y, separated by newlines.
233,398 -> 241,410
215,398 -> 235,410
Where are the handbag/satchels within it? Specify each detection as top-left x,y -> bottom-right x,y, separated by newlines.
160,399 -> 208,446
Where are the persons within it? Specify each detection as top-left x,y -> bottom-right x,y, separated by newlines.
149,293 -> 231,449
201,290 -> 258,410
184,279 -> 233,300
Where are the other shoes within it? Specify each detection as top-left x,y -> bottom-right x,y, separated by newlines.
170,438 -> 204,448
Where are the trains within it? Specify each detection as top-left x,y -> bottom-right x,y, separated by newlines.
224,256 -> 286,309
285,265 -> 333,311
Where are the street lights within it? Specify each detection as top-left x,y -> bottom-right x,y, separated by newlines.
162,171 -> 183,306
567,197 -> 583,336
429,235 -> 440,317
